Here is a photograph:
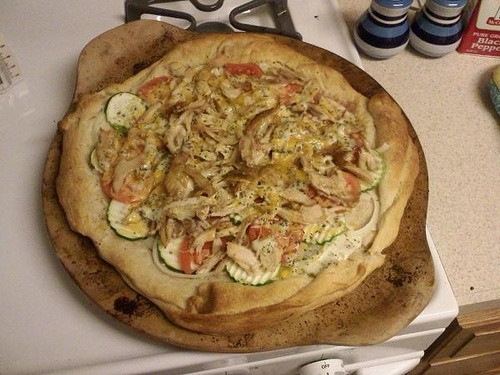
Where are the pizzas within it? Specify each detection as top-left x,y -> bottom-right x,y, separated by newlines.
56,32 -> 420,336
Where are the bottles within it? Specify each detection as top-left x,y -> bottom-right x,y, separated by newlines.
353,0 -> 413,60
408,0 -> 467,57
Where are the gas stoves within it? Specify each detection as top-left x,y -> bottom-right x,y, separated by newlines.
0,0 -> 460,375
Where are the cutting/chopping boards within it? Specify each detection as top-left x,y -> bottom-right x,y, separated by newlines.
40,17 -> 441,352
